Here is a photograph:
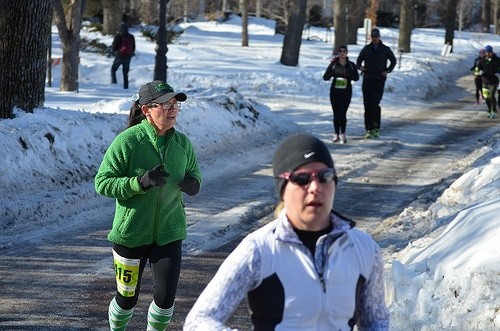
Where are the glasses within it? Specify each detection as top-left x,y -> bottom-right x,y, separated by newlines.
338,50 -> 346,52
146,102 -> 181,109
278,167 -> 336,186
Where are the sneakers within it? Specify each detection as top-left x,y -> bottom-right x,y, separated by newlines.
365,131 -> 373,138
373,130 -> 380,137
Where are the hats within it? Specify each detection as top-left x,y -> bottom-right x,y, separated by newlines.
339,45 -> 348,52
370,29 -> 380,38
271,134 -> 336,200
138,80 -> 187,107
485,45 -> 492,52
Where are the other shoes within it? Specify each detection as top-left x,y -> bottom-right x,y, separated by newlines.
338,134 -> 347,145
487,112 -> 491,118
480,94 -> 484,99
333,134 -> 338,142
476,100 -> 481,105
492,111 -> 495,117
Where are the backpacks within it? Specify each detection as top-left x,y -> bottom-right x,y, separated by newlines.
119,35 -> 132,53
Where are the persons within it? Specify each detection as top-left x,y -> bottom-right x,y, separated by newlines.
324,45 -> 359,144
95,81 -> 202,331
183,133 -> 389,331
470,45 -> 500,118
356,29 -> 397,138
107,22 -> 135,89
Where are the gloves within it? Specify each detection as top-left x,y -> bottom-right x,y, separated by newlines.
137,163 -> 169,193
178,172 -> 200,196
